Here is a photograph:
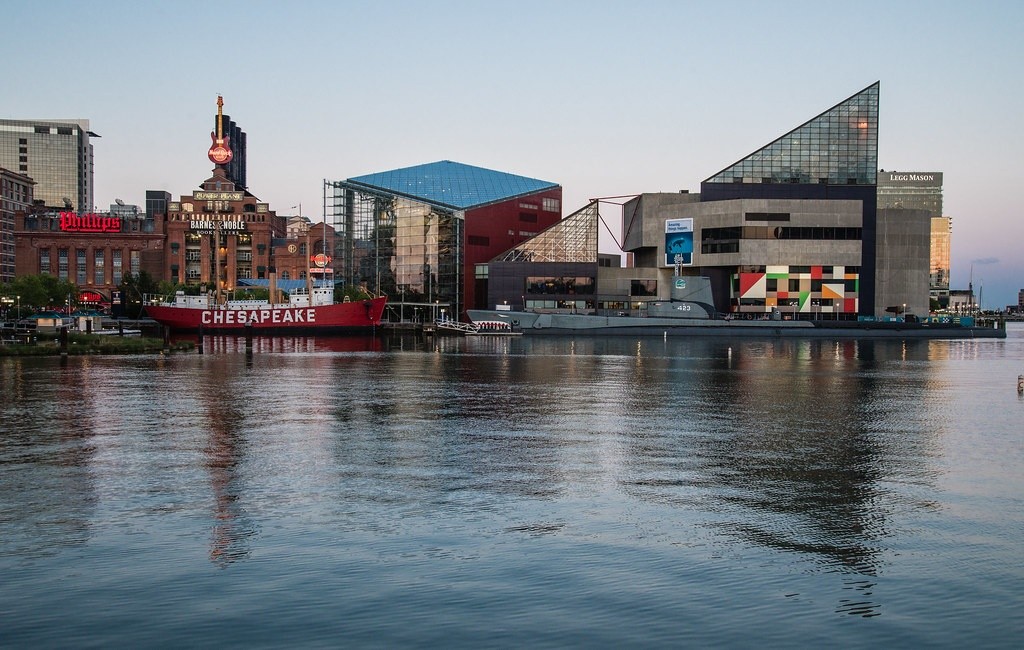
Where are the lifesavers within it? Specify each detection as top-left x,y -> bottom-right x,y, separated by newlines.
512,318 -> 520,326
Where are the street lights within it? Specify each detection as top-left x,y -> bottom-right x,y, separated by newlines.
962,305 -> 965,317
17,295 -> 21,320
968,304 -> 971,318
811,301 -> 820,321
902,303 -> 907,323
974,303 -> 978,327
84,296 -> 88,311
836,303 -> 840,321
790,302 -> 799,321
957,306 -> 960,314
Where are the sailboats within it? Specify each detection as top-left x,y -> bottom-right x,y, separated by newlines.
141,199 -> 388,334
